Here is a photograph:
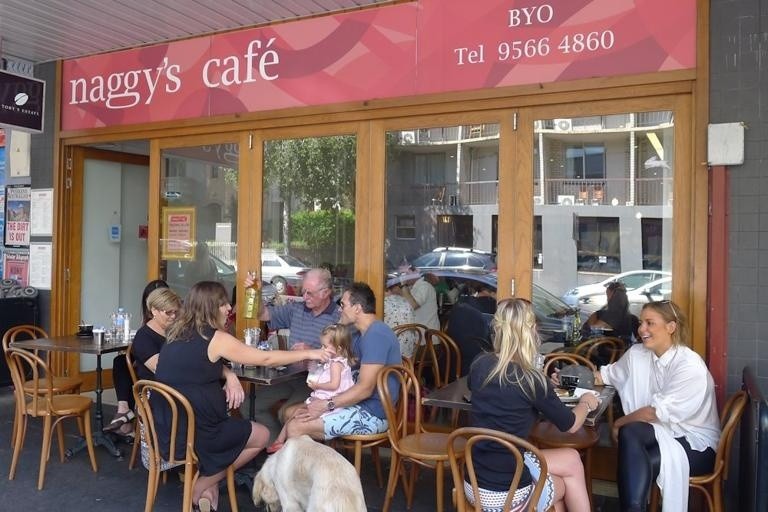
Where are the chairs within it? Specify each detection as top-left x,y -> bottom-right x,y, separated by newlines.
443,427 -> 549,510
572,335 -> 627,441
130,378 -> 239,511
533,353 -> 601,498
650,392 -> 750,511
425,330 -> 462,426
3,321 -> 106,511
377,363 -> 464,510
123,343 -> 200,484
330,355 -> 413,492
590,182 -> 605,206
576,183 -> 589,206
392,322 -> 428,377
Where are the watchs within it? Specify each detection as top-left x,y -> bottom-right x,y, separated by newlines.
328,397 -> 335,412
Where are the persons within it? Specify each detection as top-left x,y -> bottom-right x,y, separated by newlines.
462,298 -> 592,512
262,323 -> 356,453
129,285 -> 184,390
242,267 -> 357,447
183,242 -> 220,282
139,280 -> 337,512
286,279 -> 403,441
579,282 -> 642,363
385,255 -> 497,382
102,280 -> 169,434
548,299 -> 723,512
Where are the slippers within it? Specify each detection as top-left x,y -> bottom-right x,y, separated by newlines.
196,496 -> 216,511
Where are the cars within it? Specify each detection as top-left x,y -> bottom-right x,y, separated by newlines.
562,269 -> 673,306
233,249 -> 307,293
578,250 -> 664,271
395,246 -> 497,272
158,237 -> 284,304
334,268 -> 589,342
576,276 -> 673,326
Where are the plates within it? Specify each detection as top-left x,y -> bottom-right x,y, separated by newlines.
552,388 -> 602,404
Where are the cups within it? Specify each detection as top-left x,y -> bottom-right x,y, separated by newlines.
561,375 -> 580,396
77,325 -> 104,346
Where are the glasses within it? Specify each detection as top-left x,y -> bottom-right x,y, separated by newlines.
163,309 -> 179,316
300,286 -> 328,296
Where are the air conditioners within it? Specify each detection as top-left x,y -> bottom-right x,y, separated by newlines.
554,119 -> 574,133
533,196 -> 543,205
557,194 -> 575,207
401,131 -> 416,145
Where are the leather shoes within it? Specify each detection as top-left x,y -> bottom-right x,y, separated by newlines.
102,408 -> 137,445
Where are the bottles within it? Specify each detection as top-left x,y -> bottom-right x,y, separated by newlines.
106,307 -> 131,343
243,269 -> 260,320
232,326 -> 272,369
572,309 -> 582,343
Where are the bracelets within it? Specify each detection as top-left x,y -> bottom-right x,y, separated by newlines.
580,401 -> 591,413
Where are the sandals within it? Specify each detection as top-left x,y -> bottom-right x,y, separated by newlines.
265,438 -> 286,454
